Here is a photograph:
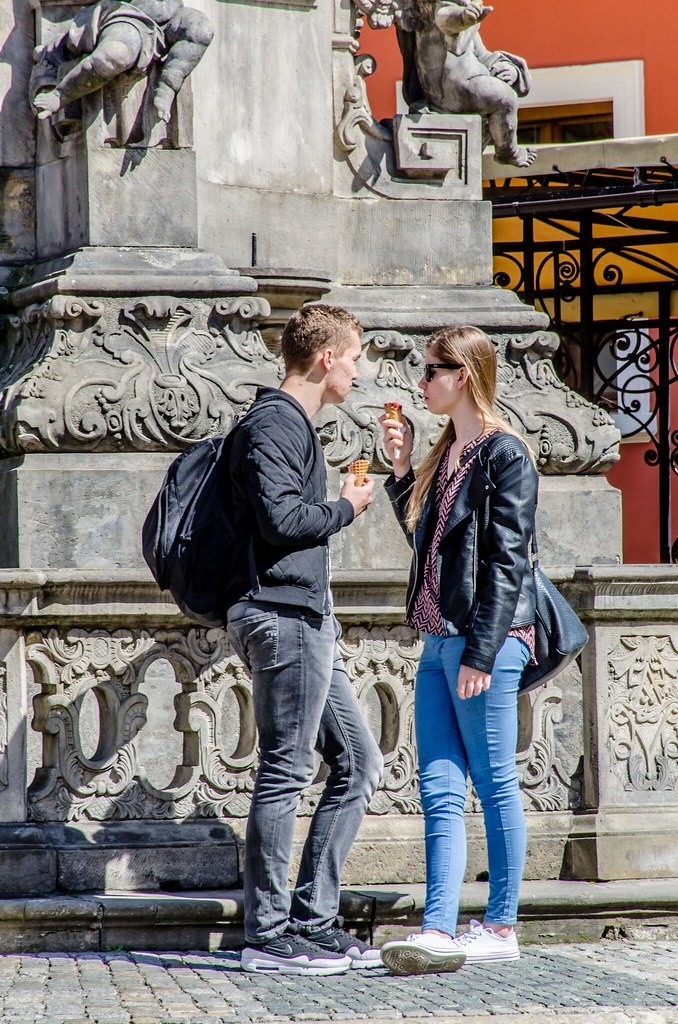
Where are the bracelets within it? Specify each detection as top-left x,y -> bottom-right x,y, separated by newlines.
395,475 -> 402,479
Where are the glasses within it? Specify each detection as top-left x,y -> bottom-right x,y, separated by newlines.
423,362 -> 466,383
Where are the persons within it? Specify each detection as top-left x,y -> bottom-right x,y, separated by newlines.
218,305 -> 392,977
31,0 -> 214,123
389,1 -> 539,169
378,327 -> 541,977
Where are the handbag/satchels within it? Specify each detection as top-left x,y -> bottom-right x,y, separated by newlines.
517,512 -> 590,697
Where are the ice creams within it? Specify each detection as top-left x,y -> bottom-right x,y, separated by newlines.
347,460 -> 370,487
383,401 -> 402,423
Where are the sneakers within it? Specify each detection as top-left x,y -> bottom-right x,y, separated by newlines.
451,918 -> 520,964
240,930 -> 353,976
380,932 -> 466,975
307,927 -> 386,967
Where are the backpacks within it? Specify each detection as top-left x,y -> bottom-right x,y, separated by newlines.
141,398 -> 314,628
